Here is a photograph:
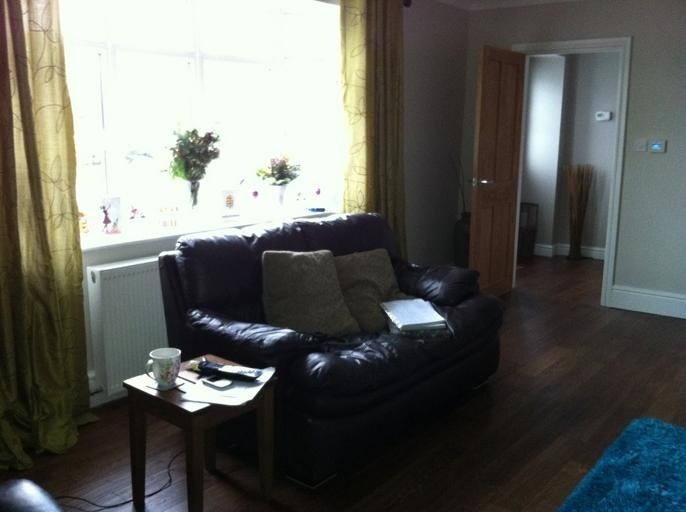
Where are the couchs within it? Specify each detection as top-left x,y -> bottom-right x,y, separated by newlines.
158,210 -> 506,497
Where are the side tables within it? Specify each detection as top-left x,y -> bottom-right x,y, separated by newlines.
121,354 -> 275,512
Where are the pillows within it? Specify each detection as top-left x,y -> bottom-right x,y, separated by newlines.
260,244 -> 408,340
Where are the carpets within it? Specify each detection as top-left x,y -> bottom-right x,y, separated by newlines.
555,416 -> 686,512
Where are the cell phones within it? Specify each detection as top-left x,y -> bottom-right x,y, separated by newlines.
201,375 -> 233,389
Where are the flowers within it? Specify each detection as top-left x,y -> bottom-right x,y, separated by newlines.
167,125 -> 221,182
257,153 -> 304,188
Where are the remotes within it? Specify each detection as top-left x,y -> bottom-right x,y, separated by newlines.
198,361 -> 263,380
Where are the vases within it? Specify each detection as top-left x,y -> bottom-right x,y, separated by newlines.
453,211 -> 471,268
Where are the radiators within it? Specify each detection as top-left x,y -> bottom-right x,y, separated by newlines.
86,254 -> 169,397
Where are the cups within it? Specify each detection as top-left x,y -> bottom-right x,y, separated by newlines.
144,347 -> 181,386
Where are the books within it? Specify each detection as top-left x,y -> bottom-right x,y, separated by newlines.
380,298 -> 456,340
